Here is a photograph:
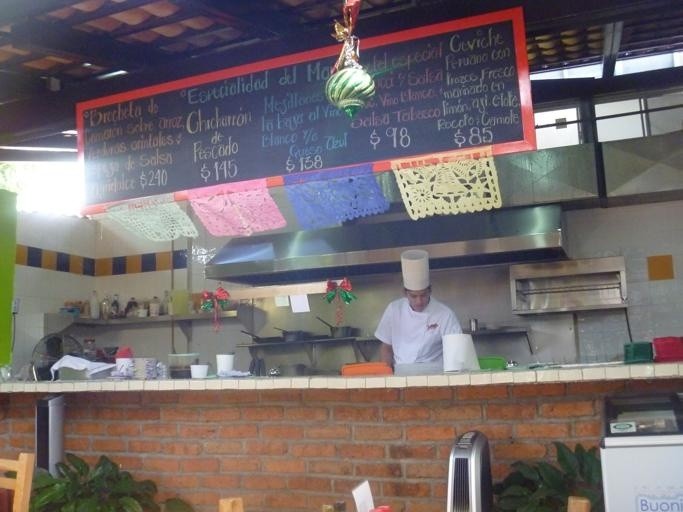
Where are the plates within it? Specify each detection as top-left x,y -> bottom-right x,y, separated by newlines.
107,375 -> 128,380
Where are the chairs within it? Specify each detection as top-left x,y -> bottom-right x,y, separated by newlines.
568,496 -> 591,512
0,452 -> 36,512
218,497 -> 244,512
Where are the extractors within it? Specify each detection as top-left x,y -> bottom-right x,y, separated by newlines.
504,255 -> 629,315
204,203 -> 570,287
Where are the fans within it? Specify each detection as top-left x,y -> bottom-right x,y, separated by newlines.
30,332 -> 83,382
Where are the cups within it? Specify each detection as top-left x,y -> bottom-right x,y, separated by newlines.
110,370 -> 120,377
479,322 -> 488,331
190,364 -> 209,379
216,354 -> 235,376
470,318 -> 478,333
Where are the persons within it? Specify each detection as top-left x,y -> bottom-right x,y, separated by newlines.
372,251 -> 463,377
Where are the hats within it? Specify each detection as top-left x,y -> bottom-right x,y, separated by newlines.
401,249 -> 430,292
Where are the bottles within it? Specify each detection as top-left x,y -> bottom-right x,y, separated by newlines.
100,295 -> 111,320
111,294 -> 120,318
126,297 -> 138,318
149,296 -> 160,316
90,289 -> 99,319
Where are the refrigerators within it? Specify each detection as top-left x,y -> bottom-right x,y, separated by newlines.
597,387 -> 683,512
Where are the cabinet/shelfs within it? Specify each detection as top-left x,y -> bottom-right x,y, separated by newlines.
236,335 -> 363,365
44,298 -> 255,336
355,326 -> 536,363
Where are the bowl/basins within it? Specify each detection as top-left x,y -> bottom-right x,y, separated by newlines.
278,364 -> 305,376
167,352 -> 200,380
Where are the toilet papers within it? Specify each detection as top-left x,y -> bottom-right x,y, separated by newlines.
441,333 -> 479,371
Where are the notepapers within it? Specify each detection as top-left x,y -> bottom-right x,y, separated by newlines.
288,294 -> 308,312
273,295 -> 288,308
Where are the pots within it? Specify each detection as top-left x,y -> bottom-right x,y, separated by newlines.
273,326 -> 304,342
315,316 -> 353,339
240,330 -> 283,344
